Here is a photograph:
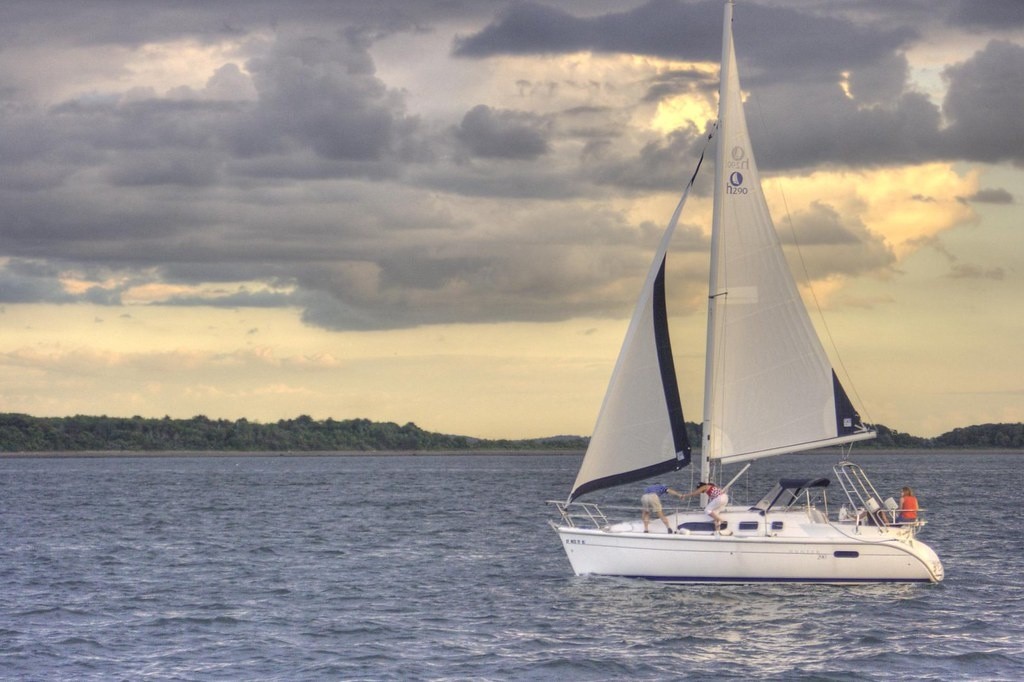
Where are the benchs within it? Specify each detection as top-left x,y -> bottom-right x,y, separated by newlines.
878,509 -> 928,527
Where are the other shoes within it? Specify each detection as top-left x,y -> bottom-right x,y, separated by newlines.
668,528 -> 673,534
644,530 -> 649,533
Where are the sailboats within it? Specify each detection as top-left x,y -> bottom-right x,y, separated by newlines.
545,1 -> 947,587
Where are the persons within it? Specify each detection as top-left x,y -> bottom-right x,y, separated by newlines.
641,482 -> 682,533
889,485 -> 919,528
680,482 -> 729,535
855,509 -> 890,527
